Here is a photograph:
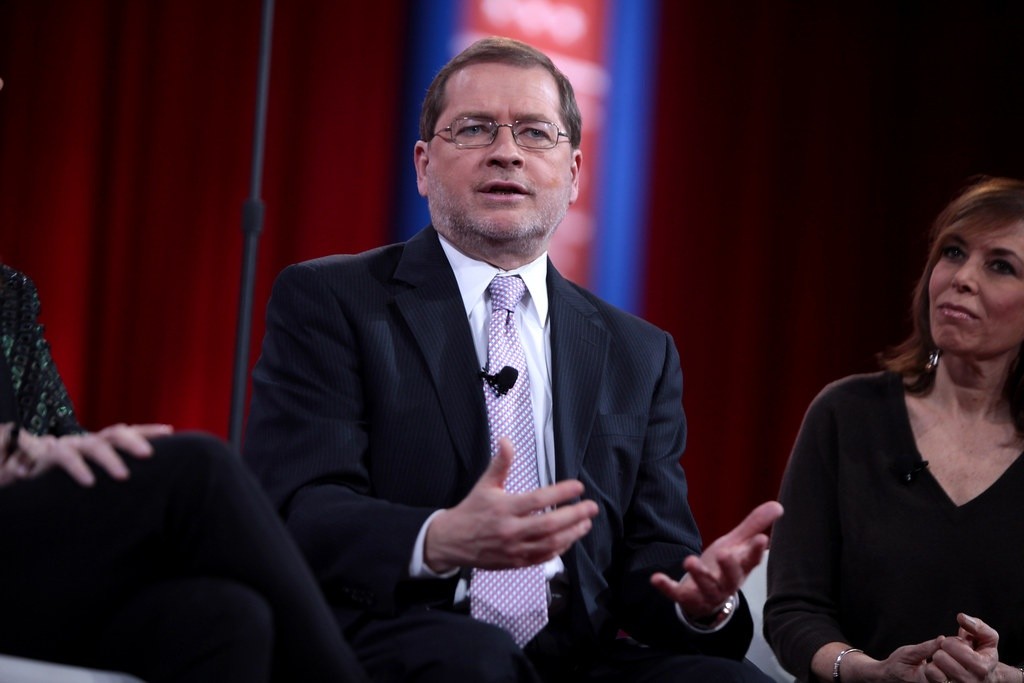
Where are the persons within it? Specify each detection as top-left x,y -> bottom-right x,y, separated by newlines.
238,36 -> 783,683
761,177 -> 1024,683
0,259 -> 370,683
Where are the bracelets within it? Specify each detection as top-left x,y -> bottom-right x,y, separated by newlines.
828,646 -> 868,683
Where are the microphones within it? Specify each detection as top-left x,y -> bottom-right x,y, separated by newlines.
493,366 -> 519,397
897,453 -> 921,483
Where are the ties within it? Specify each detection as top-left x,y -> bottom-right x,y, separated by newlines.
466,275 -> 549,649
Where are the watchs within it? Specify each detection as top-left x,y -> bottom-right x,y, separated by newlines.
696,591 -> 741,631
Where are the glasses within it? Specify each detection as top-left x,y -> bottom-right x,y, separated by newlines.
435,115 -> 571,150
